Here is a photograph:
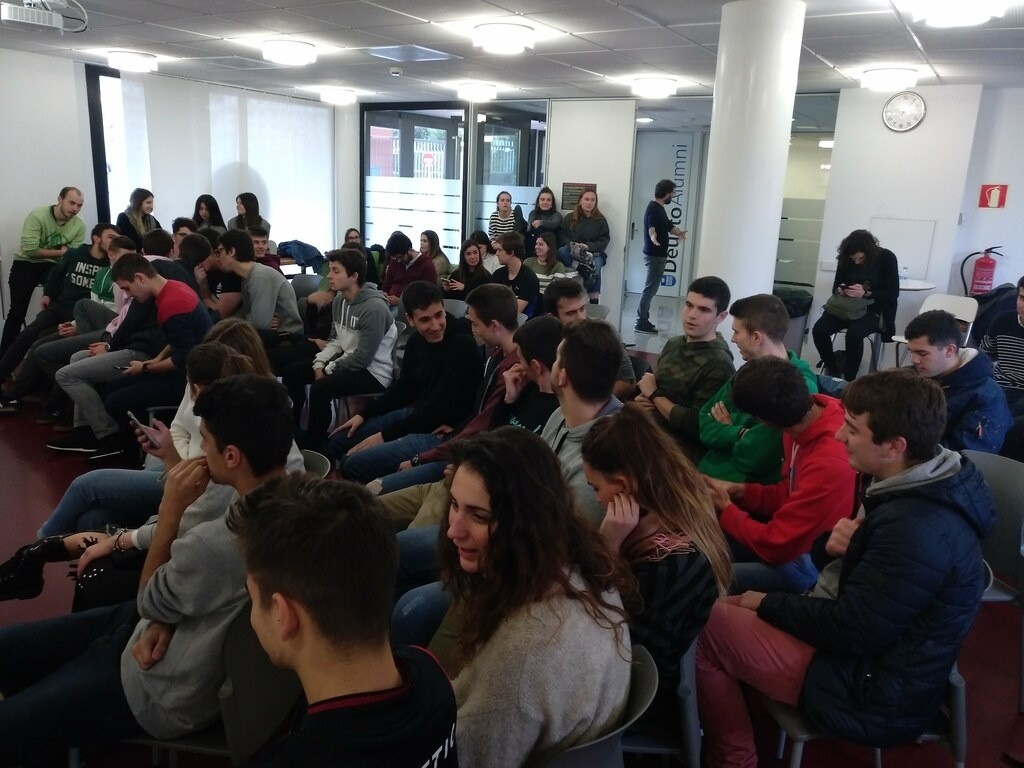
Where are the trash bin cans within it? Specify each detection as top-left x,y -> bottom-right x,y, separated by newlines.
772,287 -> 813,360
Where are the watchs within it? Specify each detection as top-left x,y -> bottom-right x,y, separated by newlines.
649,389 -> 665,400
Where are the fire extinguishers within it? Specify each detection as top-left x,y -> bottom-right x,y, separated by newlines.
960,245 -> 1003,297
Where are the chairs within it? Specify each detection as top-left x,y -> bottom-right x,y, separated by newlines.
956,449 -> 1024,602
892,294 -> 978,368
778,559 -> 993,768
143,298 -> 611,481
552,625 -> 703,768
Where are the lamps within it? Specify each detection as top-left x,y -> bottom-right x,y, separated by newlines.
632,78 -> 678,99
458,84 -> 496,102
321,89 -> 356,106
861,68 -> 919,92
473,23 -> 535,54
263,41 -> 316,65
108,51 -> 158,72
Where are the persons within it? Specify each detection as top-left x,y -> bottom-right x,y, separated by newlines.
583,274 -> 1009,768
1,187 -> 634,767
634,179 -> 687,334
812,231 -> 899,380
977,276 -> 1024,416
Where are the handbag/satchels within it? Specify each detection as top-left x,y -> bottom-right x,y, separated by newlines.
571,245 -> 596,279
821,293 -> 874,321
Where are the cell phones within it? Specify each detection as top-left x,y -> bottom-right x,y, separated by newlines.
114,366 -> 132,369
840,284 -> 850,291
441,278 -> 452,284
127,411 -> 161,449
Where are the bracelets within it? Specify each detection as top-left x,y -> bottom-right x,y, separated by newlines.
113,533 -> 126,552
410,454 -> 419,467
142,365 -> 149,372
741,428 -> 746,435
105,344 -> 110,351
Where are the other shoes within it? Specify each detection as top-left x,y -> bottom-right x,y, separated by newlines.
1,375 -> 13,392
54,411 -> 74,432
0,556 -> 45,603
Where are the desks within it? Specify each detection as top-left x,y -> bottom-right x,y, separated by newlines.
868,279 -> 936,375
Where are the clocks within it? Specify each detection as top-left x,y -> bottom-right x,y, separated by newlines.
883,91 -> 926,132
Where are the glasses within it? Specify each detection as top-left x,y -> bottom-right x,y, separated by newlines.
672,191 -> 676,196
175,232 -> 188,237
214,247 -> 238,258
348,236 -> 360,239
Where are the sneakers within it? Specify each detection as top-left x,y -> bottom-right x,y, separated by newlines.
37,406 -> 65,424
634,318 -> 658,334
0,396 -> 19,415
46,433 -> 98,452
88,435 -> 126,459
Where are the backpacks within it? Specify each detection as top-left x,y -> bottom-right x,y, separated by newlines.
968,283 -> 1019,345
